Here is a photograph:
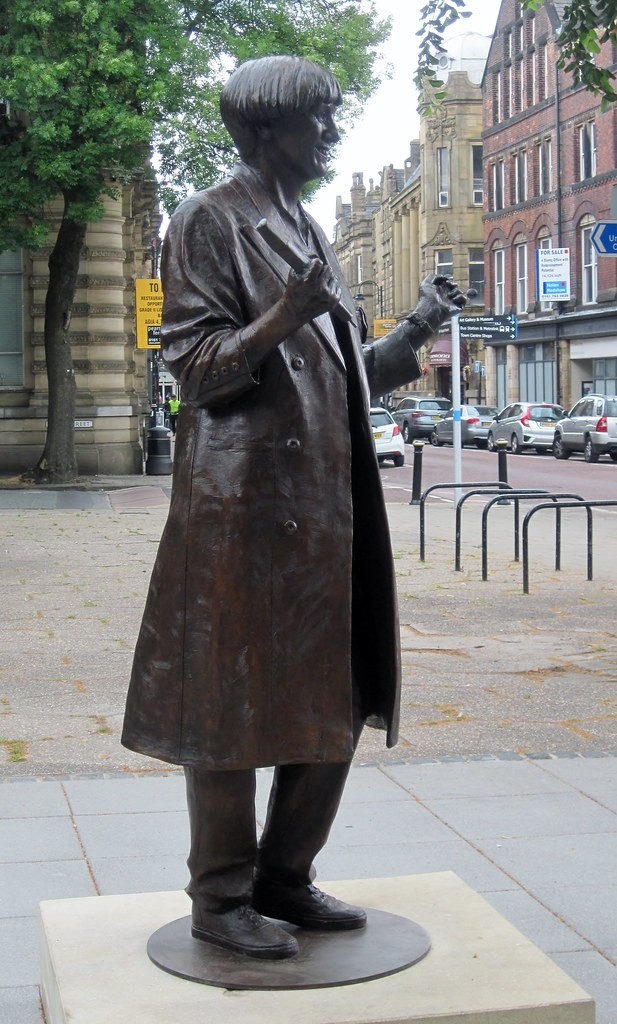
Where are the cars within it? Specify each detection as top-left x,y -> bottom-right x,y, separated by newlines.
486,402 -> 567,454
430,405 -> 499,449
370,406 -> 404,466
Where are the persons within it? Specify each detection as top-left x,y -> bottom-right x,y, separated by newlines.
168,395 -> 180,436
121,57 -> 471,957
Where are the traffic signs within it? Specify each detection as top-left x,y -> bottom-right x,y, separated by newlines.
458,315 -> 516,341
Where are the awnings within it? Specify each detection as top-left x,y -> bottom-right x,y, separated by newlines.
430,336 -> 469,368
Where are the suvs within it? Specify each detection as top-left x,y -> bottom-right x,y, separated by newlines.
389,396 -> 451,444
553,394 -> 617,464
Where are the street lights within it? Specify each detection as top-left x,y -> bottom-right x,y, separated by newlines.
354,280 -> 388,409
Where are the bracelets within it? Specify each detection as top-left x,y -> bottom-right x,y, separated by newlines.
407,312 -> 434,338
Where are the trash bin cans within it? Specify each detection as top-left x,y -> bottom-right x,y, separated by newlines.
146,426 -> 173,475
156,412 -> 164,427
